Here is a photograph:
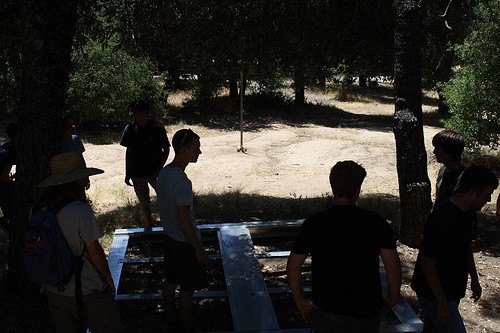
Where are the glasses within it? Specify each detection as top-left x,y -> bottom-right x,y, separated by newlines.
181,129 -> 191,145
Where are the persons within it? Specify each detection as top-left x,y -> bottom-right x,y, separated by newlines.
0,125 -> 22,223
411,164 -> 499,333
61,115 -> 90,201
28,153 -> 121,333
287,161 -> 403,333
496,194 -> 500,225
120,100 -> 171,243
155,129 -> 209,330
431,131 -> 465,216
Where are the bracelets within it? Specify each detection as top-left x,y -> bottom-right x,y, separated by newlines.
496,211 -> 500,216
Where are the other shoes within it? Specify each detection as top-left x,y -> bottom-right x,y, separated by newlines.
165,321 -> 195,333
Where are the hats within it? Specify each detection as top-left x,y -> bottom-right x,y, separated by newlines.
37,152 -> 105,187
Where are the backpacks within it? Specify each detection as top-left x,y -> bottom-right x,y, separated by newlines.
18,197 -> 86,313
